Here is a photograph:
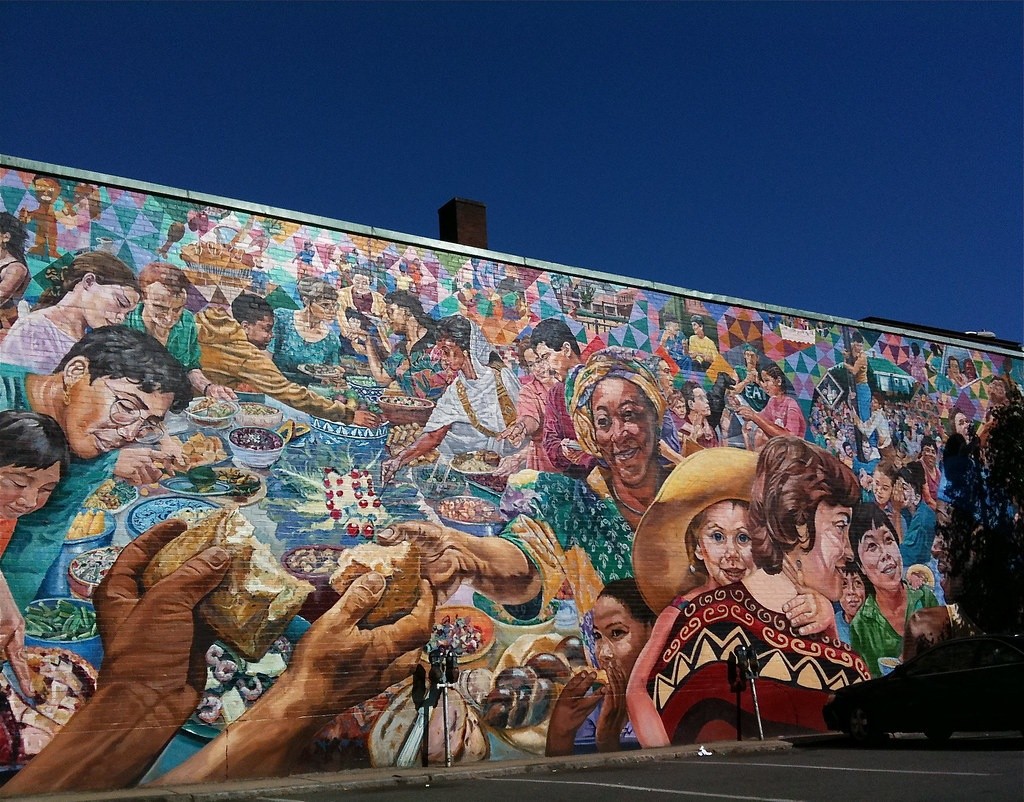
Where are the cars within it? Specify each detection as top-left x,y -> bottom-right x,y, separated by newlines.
821,634 -> 1023,749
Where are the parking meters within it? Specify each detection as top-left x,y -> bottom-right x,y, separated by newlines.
431,649 -> 458,768
738,645 -> 765,739
